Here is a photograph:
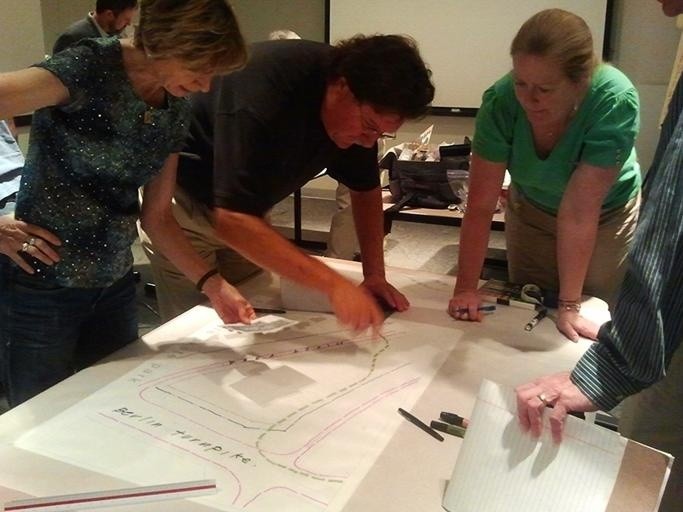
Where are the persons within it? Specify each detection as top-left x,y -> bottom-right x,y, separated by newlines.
508,1 -> 683,453
0,1 -> 259,416
50,1 -> 140,61
132,30 -> 438,344
442,6 -> 644,351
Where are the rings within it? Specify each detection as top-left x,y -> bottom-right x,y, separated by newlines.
29,237 -> 36,246
537,393 -> 548,406
21,243 -> 29,253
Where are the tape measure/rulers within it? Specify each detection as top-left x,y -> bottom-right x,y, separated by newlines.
4,479 -> 216,512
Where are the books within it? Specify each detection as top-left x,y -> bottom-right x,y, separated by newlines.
440,377 -> 680,511
477,277 -> 545,313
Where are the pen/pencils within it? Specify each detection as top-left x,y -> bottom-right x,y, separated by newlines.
525,309 -> 547,331
456,305 -> 496,312
399,408 -> 469,441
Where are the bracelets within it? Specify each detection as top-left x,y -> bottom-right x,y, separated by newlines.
555,296 -> 583,314
195,266 -> 219,295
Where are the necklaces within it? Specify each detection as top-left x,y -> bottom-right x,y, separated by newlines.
139,95 -> 158,124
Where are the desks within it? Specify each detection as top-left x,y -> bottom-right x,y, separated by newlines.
380,185 -> 510,270
0,254 -> 682,512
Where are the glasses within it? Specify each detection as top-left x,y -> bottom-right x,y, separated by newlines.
356,105 -> 398,141
